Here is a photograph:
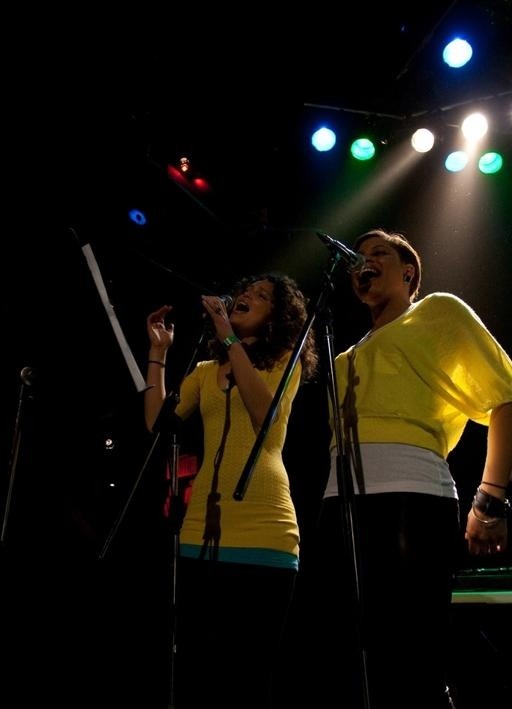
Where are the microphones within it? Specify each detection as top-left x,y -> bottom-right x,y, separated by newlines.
201,291 -> 235,316
20,365 -> 36,402
318,229 -> 367,272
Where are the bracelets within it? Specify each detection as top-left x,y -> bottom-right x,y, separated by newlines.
147,360 -> 165,367
470,480 -> 512,527
223,335 -> 240,349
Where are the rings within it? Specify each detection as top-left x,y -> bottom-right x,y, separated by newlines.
216,307 -> 222,313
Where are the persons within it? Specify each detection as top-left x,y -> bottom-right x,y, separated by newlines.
140,274 -> 320,708
314,229 -> 512,709
